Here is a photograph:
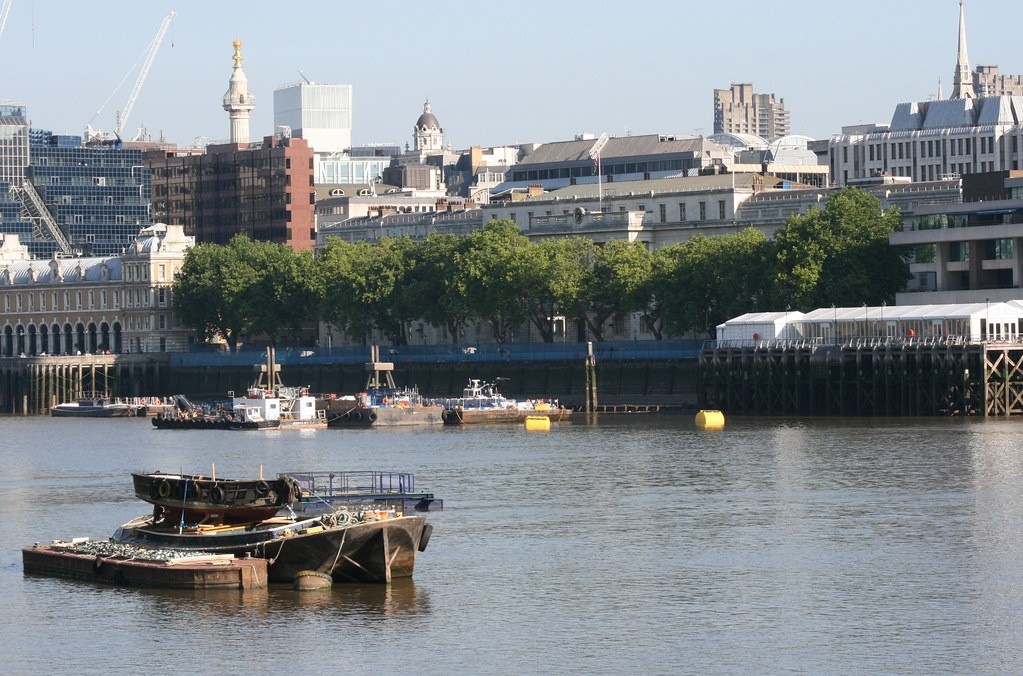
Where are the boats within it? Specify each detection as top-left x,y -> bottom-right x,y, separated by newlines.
130,468 -> 301,523
50,396 -> 149,418
151,405 -> 281,430
459,407 -> 573,424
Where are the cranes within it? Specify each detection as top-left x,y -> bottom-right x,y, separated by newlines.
82,7 -> 177,146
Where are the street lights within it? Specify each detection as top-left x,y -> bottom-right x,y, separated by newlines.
830,302 -> 838,346
986,297 -> 991,341
862,301 -> 868,345
85,328 -> 89,352
785,304 -> 792,348
20,330 -> 24,354
418,323 -> 424,345
881,300 -> 887,347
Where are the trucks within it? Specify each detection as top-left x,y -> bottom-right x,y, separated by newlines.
300,351 -> 314,357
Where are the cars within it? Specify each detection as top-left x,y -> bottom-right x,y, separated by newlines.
389,349 -> 399,355
462,346 -> 477,354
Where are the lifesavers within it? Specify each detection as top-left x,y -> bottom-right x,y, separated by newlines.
255,480 -> 270,495
368,412 -> 378,422
418,523 -> 433,552
151,417 -> 221,429
293,481 -> 303,499
207,485 -> 225,504
172,485 -> 183,500
331,411 -> 363,423
149,483 -> 159,500
159,481 -> 171,498
188,484 -> 202,501
